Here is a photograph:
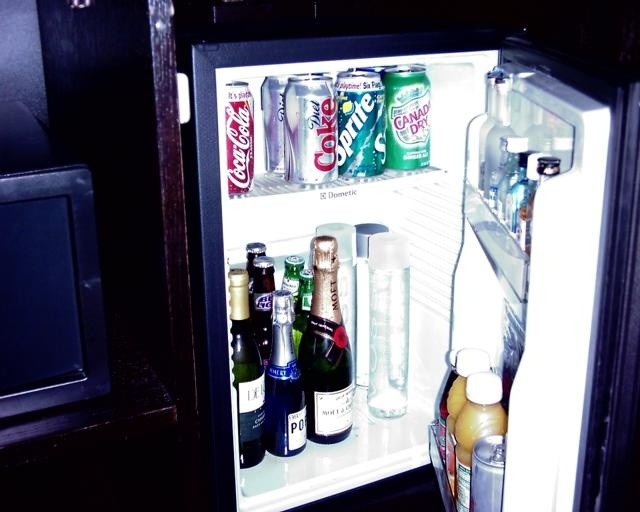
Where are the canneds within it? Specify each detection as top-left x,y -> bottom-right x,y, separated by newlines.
223,63 -> 432,196
472,434 -> 507,512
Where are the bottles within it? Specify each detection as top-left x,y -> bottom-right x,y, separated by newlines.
227,219 -> 412,470
476,68 -> 563,260
428,346 -> 506,512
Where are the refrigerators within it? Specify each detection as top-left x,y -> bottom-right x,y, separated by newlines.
188,27 -> 640,512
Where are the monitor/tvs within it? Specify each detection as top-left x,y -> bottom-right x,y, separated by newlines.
0,164 -> 111,419
